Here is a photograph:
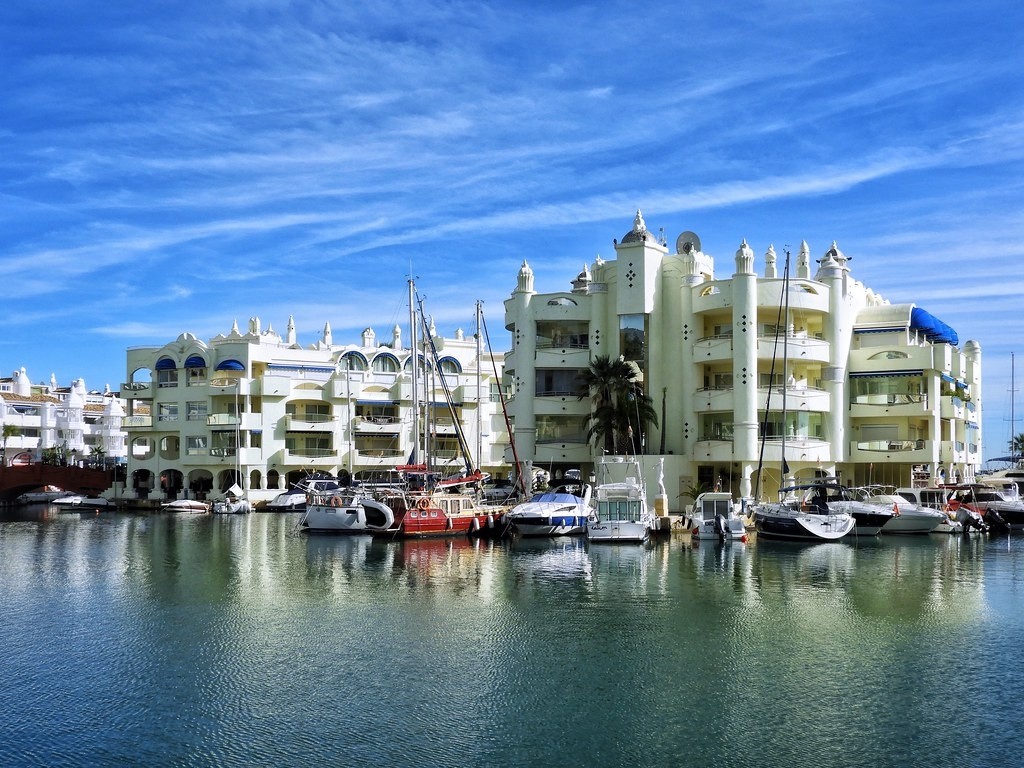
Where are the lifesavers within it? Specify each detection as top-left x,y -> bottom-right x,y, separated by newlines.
419,498 -> 430,511
330,497 -> 343,506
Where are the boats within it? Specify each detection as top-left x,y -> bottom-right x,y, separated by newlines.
361,266 -> 531,538
52,496 -> 110,510
161,498 -> 212,513
848,483 -> 946,533
588,446 -> 655,542
777,482 -> 897,537
211,379 -> 251,514
265,470 -> 348,510
688,490 -> 747,540
893,354 -> 1024,533
306,358 -> 367,533
506,478 -> 592,537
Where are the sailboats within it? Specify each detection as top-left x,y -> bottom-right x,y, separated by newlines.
750,251 -> 857,540
